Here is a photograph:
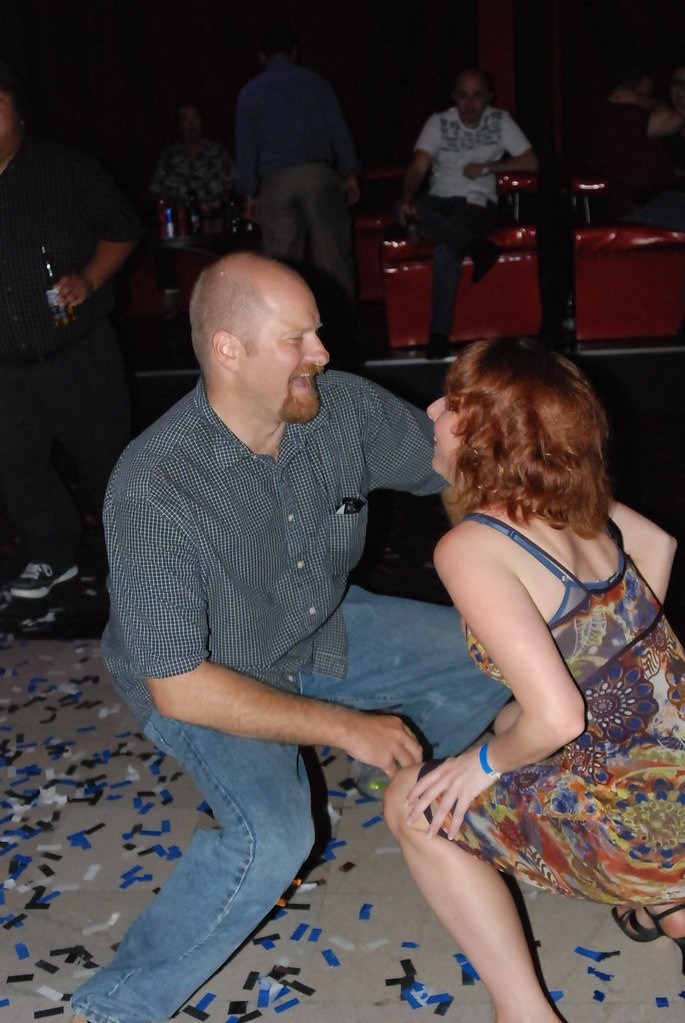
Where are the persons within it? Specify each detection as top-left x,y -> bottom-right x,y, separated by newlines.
69,252 -> 513,1023
151,98 -> 238,323
0,62 -> 148,599
580,46 -> 685,236
236,28 -> 361,363
395,66 -> 540,388
382,338 -> 685,1022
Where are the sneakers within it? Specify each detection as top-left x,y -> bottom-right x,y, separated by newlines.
12,560 -> 78,597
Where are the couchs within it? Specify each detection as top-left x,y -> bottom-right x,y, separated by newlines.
126,162 -> 542,349
573,174 -> 685,341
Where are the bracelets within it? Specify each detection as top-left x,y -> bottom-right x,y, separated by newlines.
479,743 -> 502,779
78,267 -> 96,301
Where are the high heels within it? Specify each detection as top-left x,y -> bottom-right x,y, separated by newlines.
612,903 -> 685,975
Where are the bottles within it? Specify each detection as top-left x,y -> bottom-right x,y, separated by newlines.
225,188 -> 242,236
166,207 -> 174,238
159,201 -> 166,239
41,252 -> 78,330
186,190 -> 200,235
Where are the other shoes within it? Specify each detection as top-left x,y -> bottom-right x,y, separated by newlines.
470,238 -> 499,283
426,333 -> 450,360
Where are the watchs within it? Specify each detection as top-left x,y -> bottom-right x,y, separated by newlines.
482,164 -> 490,175
